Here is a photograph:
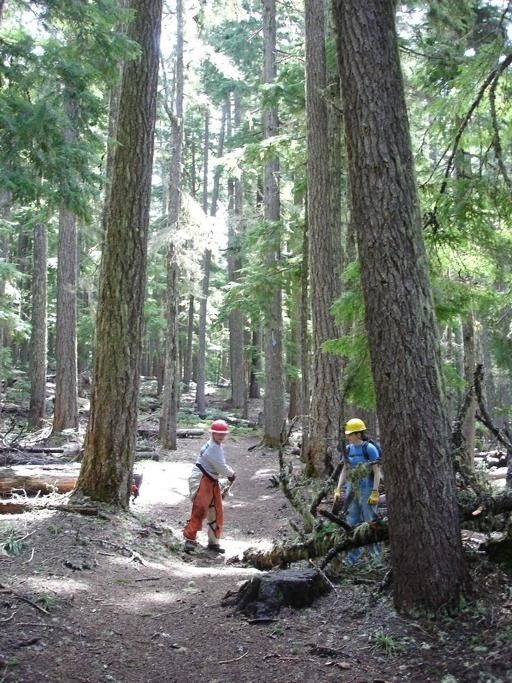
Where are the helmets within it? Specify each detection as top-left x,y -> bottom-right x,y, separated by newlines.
345,419 -> 367,434
209,420 -> 229,433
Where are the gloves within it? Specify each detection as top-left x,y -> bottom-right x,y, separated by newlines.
228,474 -> 236,481
335,488 -> 340,502
368,491 -> 379,505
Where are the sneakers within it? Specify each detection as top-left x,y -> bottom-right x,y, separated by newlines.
208,543 -> 225,553
185,541 -> 195,551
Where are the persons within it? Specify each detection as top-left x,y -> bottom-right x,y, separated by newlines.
183,420 -> 233,552
334,418 -> 383,569
